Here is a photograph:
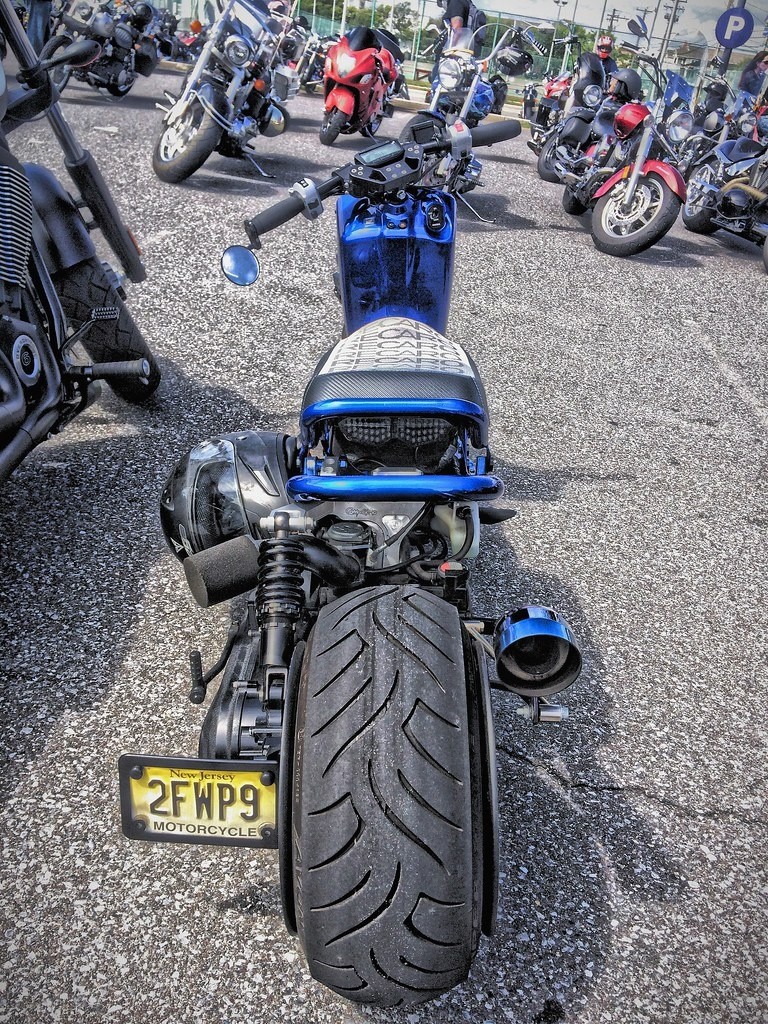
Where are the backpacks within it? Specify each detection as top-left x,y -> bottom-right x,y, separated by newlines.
463,0 -> 486,58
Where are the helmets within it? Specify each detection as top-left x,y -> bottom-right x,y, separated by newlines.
496,46 -> 533,76
159,431 -> 296,563
295,15 -> 309,25
610,69 -> 642,101
613,104 -> 651,140
130,3 -> 153,27
703,109 -> 724,132
189,20 -> 203,34
259,104 -> 285,136
596,36 -> 612,59
721,188 -> 752,218
703,82 -> 728,101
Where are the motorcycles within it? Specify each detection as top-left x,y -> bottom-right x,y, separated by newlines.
0,0 -> 162,493
0,0 -> 768,258
117,107 -> 583,1008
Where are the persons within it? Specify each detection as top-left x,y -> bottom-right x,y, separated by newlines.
428,0 -> 482,85
596,36 -> 619,73
738,51 -> 768,105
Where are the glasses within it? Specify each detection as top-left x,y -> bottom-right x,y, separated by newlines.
762,60 -> 768,65
596,44 -> 612,53
